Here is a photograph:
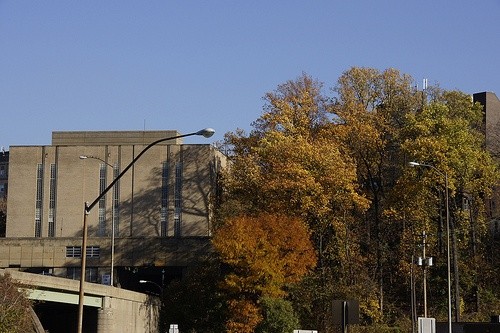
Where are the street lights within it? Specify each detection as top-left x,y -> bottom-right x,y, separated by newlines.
78,154 -> 117,286
410,161 -> 453,333
139,277 -> 168,332
75,127 -> 218,333
